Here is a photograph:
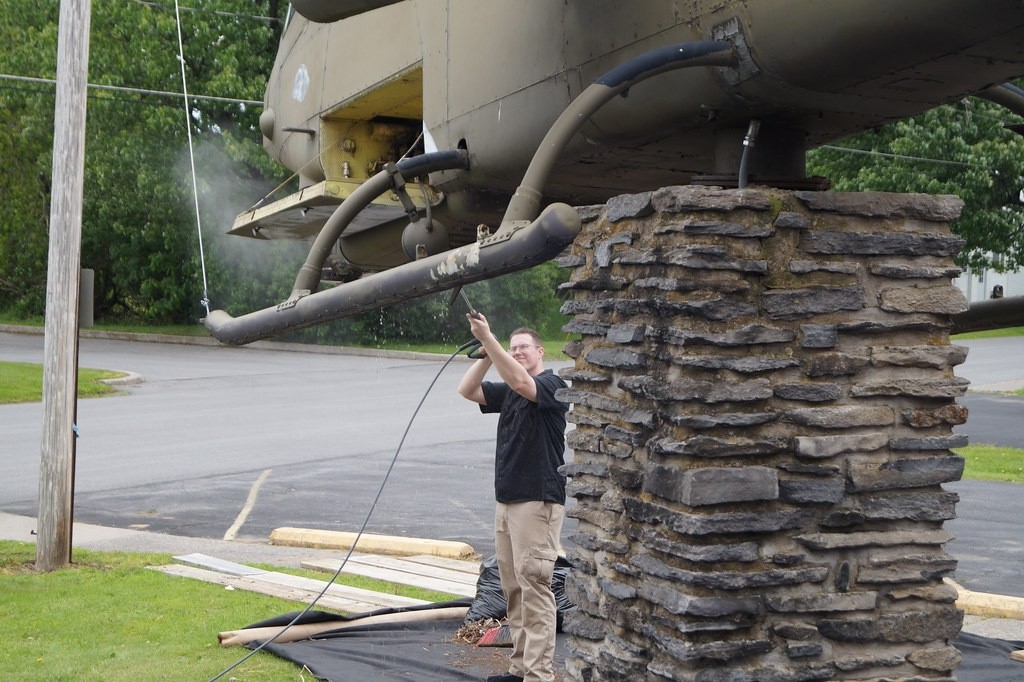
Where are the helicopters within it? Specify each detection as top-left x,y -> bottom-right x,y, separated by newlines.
202,1 -> 1024,347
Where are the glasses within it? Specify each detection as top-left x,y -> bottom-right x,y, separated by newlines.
508,344 -> 539,352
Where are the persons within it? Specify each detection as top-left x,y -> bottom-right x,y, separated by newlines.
459,312 -> 570,682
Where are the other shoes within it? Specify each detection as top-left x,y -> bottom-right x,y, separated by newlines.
487,672 -> 524,682
